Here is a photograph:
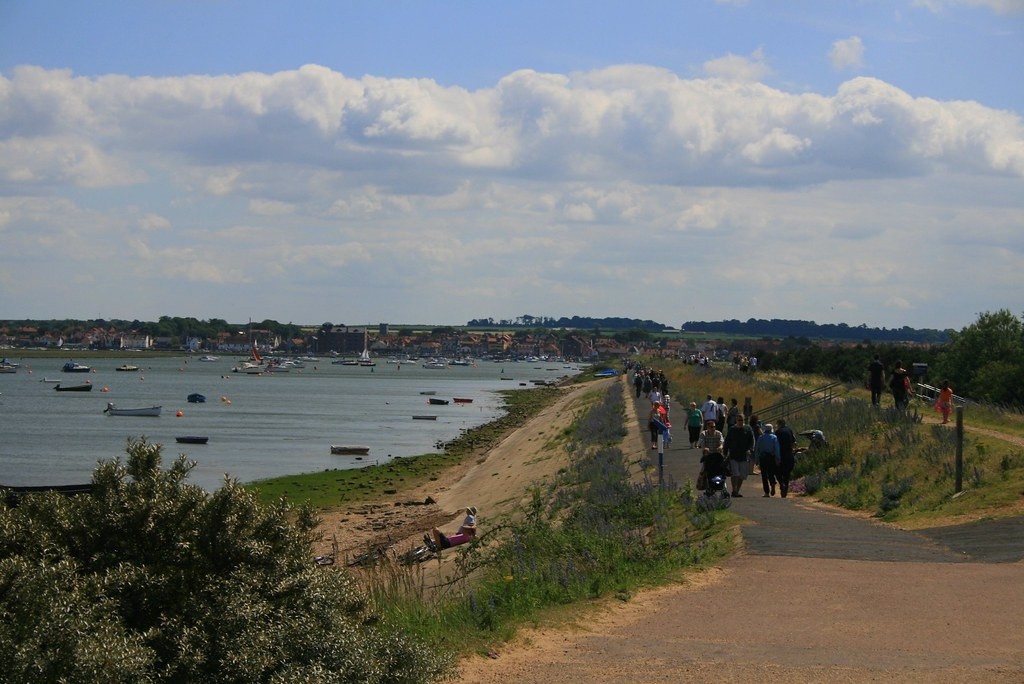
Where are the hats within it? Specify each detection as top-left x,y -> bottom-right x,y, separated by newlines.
764,424 -> 773,431
776,419 -> 786,424
467,506 -> 478,516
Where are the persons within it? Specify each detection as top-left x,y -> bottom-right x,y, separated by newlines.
938,378 -> 954,424
618,352 -> 914,497
430,506 -> 477,551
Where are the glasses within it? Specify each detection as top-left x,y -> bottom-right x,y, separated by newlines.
737,420 -> 744,421
709,426 -> 716,428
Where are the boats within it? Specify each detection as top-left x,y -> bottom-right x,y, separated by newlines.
419,390 -> 437,395
331,444 -> 370,456
482,352 -> 571,387
385,354 -> 476,370
116,364 -> 140,371
412,413 -> 438,421
62,362 -> 90,372
231,318 -> 320,375
174,435 -> 211,444
202,355 -> 218,362
0,357 -> 21,374
187,392 -> 207,403
428,398 -> 450,406
103,403 -> 163,417
332,326 -> 376,368
42,376 -> 61,384
53,384 -> 94,392
453,397 -> 474,404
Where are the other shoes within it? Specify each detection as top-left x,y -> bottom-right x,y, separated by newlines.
735,494 -> 743,497
780,484 -> 787,498
731,492 -> 736,496
762,492 -> 769,497
771,483 -> 775,495
695,443 -> 699,449
651,445 -> 657,449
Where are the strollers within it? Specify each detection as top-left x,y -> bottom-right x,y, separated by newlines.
703,451 -> 730,500
